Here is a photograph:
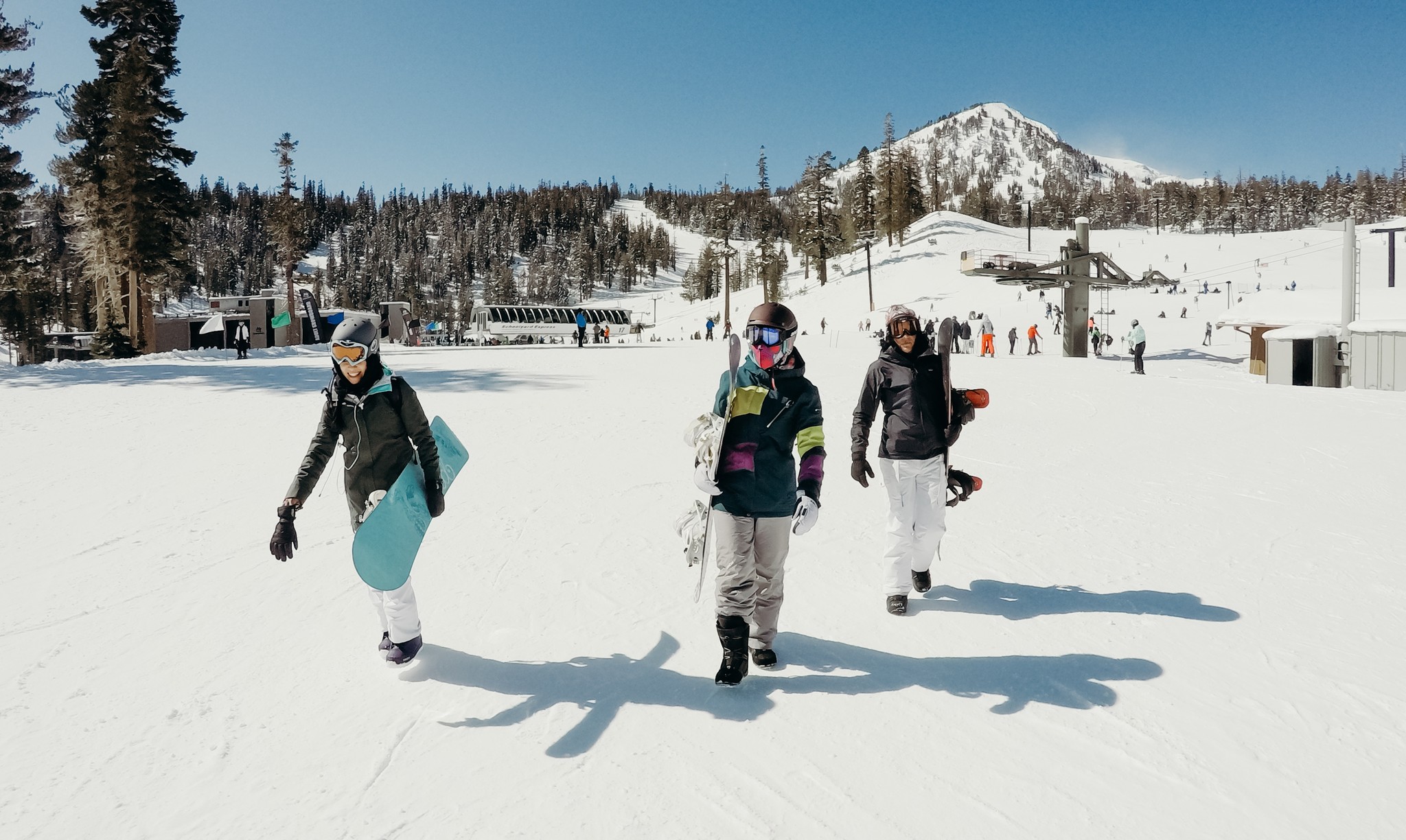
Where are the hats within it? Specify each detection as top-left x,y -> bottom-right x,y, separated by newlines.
885,304 -> 912,325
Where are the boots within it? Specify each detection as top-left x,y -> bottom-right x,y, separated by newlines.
715,614 -> 749,687
749,646 -> 777,668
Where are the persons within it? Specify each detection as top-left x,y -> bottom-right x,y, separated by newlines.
693,301 -> 827,685
850,305 -> 960,615
233,320 -> 250,360
438,254 -> 1296,374
269,316 -> 444,667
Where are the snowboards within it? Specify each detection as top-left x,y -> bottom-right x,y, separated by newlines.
693,333 -> 741,604
937,316 -> 956,562
352,415 -> 471,591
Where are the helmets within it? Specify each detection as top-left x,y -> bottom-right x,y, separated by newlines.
746,302 -> 797,369
331,317 -> 379,357
1131,320 -> 1138,326
1034,324 -> 1037,327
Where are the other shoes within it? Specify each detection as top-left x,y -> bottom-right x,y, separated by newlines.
244,356 -> 247,359
238,356 -> 241,359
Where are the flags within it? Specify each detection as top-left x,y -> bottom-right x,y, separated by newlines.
271,310 -> 291,329
198,313 -> 225,335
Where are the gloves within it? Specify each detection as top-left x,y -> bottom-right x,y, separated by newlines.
943,420 -> 962,447
694,456 -> 726,495
790,488 -> 820,535
852,452 -> 875,487
270,505 -> 298,562
426,483 -> 445,518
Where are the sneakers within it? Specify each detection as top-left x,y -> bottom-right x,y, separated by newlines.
911,569 -> 931,592
378,631 -> 394,652
386,635 -> 423,667
887,595 -> 908,614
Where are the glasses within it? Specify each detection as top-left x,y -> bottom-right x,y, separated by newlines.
747,325 -> 784,347
887,316 -> 920,338
330,340 -> 369,367
